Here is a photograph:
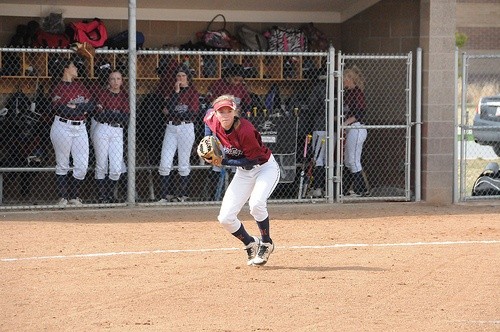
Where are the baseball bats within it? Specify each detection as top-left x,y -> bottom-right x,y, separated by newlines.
248,106 -> 267,117
298,135 -> 324,199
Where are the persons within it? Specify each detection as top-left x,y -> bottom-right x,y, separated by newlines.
90,70 -> 129,207
343,66 -> 373,197
157,67 -> 200,203
198,95 -> 280,266
207,66 -> 251,200
49,59 -> 91,206
311,66 -> 337,198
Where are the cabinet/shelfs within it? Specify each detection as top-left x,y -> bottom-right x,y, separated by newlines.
0,50 -> 323,95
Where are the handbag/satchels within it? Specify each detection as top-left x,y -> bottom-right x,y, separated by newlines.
39,30 -> 72,47
69,17 -> 108,47
8,20 -> 40,49
307,23 -> 329,52
265,24 -> 308,52
237,24 -> 268,50
196,13 -> 233,50
106,29 -> 145,49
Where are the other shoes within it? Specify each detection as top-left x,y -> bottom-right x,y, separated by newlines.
68,197 -> 83,205
56,197 -> 67,206
312,188 -> 326,197
159,195 -> 174,202
348,189 -> 371,197
170,196 -> 190,202
98,196 -> 120,203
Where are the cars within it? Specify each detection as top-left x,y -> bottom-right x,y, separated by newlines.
473,94 -> 500,157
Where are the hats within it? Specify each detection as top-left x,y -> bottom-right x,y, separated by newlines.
213,98 -> 236,111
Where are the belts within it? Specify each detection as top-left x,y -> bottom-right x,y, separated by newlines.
167,120 -> 191,126
360,121 -> 364,124
241,160 -> 268,170
94,117 -> 123,128
59,118 -> 85,125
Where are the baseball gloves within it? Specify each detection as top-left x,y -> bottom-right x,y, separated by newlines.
197,134 -> 226,167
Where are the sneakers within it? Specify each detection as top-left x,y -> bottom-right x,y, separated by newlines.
253,238 -> 274,265
244,236 -> 260,266
